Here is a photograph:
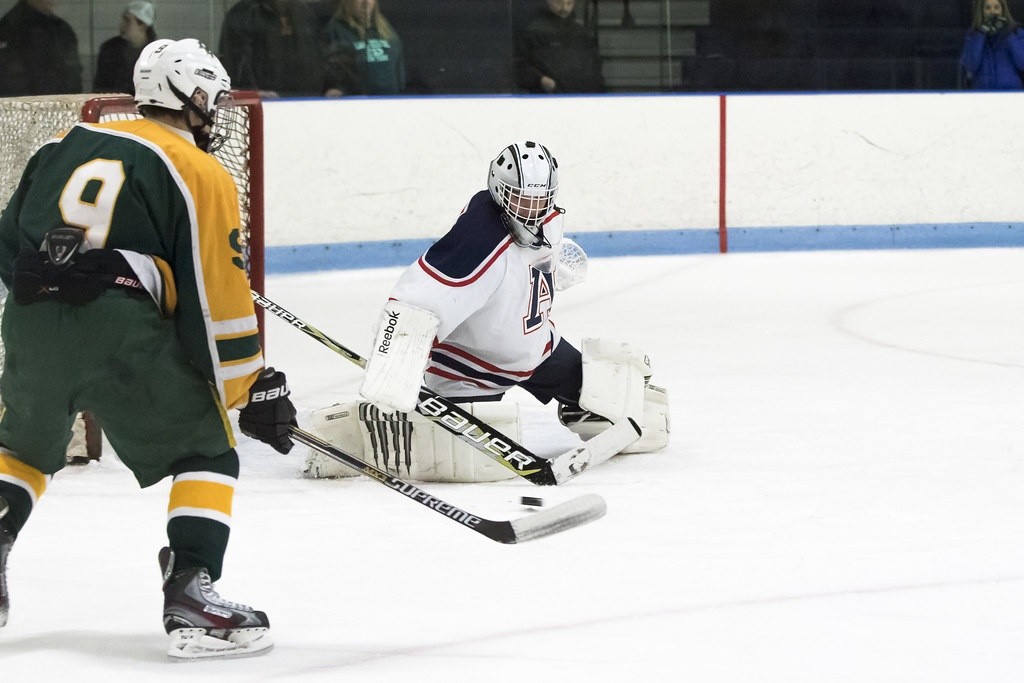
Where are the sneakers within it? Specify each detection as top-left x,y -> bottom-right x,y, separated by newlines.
0,496 -> 18,628
158,547 -> 273,658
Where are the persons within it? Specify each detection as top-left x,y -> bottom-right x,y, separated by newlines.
956,0 -> 1023,91
321,0 -> 405,98
220,0 -> 324,97
0,39 -> 295,656
92,2 -> 159,96
299,143 -> 669,482
0,0 -> 83,100
518,0 -> 608,94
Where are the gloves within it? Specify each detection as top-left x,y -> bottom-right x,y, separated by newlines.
238,367 -> 299,455
979,16 -> 996,37
993,15 -> 1007,31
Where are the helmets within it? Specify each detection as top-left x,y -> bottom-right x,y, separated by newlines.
132,38 -> 236,154
487,141 -> 560,247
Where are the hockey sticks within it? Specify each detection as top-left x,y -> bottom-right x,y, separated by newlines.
284,424 -> 607,545
248,287 -> 643,487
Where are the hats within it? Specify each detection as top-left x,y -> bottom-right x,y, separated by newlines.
126,1 -> 157,41
